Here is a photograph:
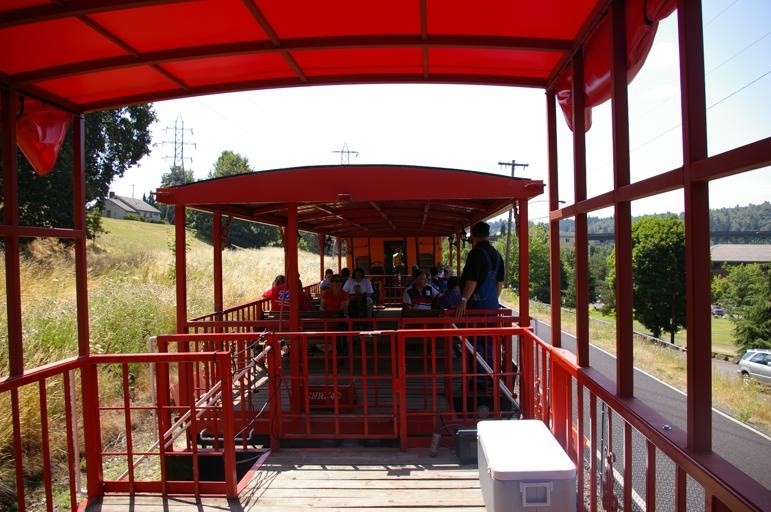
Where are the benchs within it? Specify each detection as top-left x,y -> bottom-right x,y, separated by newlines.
260,281 -> 488,361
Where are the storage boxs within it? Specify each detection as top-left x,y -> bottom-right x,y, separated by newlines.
477,420 -> 577,512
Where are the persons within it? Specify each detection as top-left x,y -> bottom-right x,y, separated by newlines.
401,264 -> 461,311
456,221 -> 504,393
392,252 -> 402,287
262,266 -> 375,318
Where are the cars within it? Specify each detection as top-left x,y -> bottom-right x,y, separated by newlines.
712,304 -> 734,316
594,299 -> 605,311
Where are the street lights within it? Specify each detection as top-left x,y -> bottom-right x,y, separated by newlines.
525,200 -> 568,204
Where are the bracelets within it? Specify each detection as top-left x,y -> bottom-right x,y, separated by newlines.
460,296 -> 468,304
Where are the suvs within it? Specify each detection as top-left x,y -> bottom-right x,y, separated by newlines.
737,347 -> 771,388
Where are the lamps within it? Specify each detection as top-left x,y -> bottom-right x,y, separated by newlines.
298,232 -> 332,253
448,229 -> 466,250
